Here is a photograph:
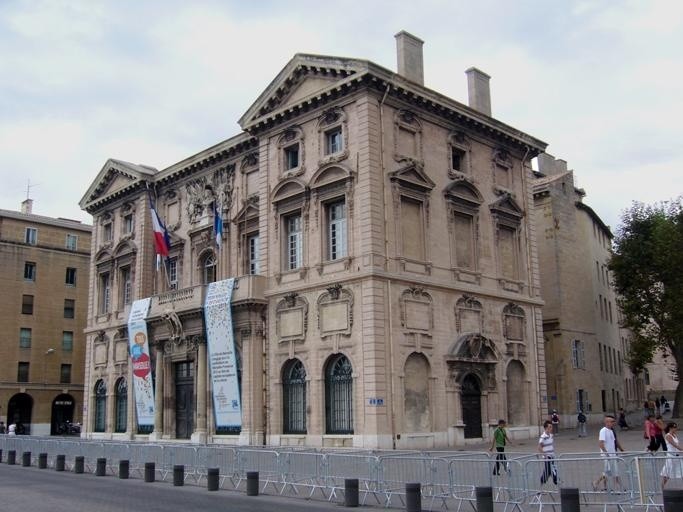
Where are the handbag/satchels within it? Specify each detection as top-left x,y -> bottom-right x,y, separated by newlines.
644,432 -> 650,439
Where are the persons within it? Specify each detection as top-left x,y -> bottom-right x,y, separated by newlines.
644,395 -> 670,415
0,421 -> 17,435
618,408 -> 632,431
591,414 -> 627,492
536,421 -> 565,487
660,422 -> 683,490
489,419 -> 513,476
645,415 -> 667,456
577,408 -> 587,438
550,409 -> 560,434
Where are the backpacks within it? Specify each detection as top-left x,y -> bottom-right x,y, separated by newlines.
552,415 -> 558,422
578,414 -> 586,423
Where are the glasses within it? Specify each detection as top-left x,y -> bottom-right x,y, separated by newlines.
671,426 -> 679,428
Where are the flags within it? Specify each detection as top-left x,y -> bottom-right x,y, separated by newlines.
212,198 -> 223,246
149,206 -> 171,256
